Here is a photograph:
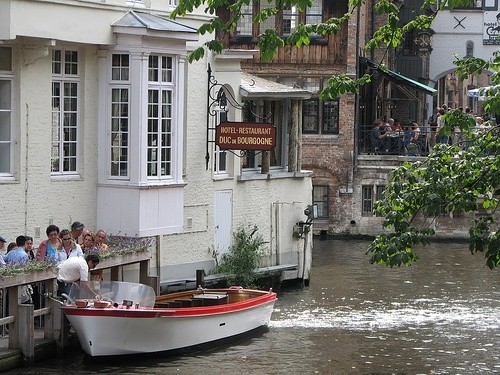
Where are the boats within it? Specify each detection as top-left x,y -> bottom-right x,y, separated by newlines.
59,282 -> 279,359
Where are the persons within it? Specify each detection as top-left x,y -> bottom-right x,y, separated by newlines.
69,222 -> 108,288
36,225 -> 63,263
369,104 -> 494,156
53,229 -> 83,265
0,235 -> 38,333
55,254 -> 100,338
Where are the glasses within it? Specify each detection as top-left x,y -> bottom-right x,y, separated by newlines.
92,262 -> 96,268
62,238 -> 71,241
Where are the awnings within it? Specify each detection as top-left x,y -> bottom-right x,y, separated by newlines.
359,55 -> 438,102
468,89 -> 480,98
